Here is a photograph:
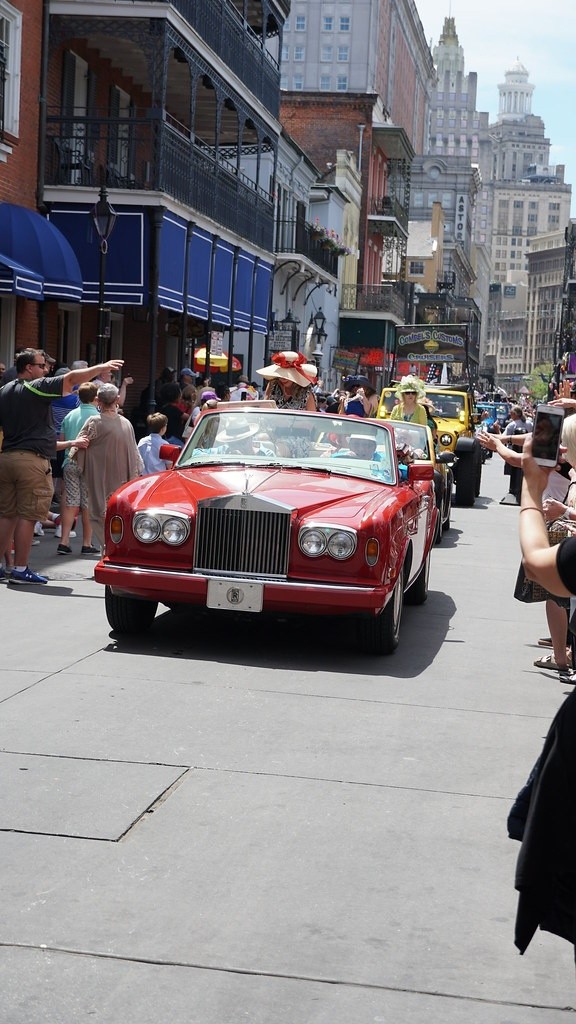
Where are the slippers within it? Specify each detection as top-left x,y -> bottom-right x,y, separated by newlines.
565,650 -> 573,669
533,651 -> 570,671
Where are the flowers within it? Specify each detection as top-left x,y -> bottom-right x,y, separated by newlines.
310,218 -> 352,255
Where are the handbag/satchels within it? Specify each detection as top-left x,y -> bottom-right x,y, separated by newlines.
182,426 -> 193,439
514,518 -> 573,603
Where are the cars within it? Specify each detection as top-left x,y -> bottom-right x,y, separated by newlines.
93,406 -> 458,656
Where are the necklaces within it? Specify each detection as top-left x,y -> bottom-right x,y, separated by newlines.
284,386 -> 292,396
102,409 -> 115,413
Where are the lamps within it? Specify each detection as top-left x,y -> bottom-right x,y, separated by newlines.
309,307 -> 327,356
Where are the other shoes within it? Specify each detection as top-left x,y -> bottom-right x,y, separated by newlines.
538,637 -> 553,646
32,540 -> 40,545
34,522 -> 45,536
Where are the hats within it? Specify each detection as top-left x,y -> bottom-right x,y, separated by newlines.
346,434 -> 377,443
39,350 -> 56,363
342,374 -> 376,395
160,367 -> 176,380
395,375 -> 426,401
214,417 -> 259,443
256,351 -> 317,387
201,391 -> 221,401
180,368 -> 196,377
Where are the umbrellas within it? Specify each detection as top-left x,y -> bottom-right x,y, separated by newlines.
194,345 -> 241,372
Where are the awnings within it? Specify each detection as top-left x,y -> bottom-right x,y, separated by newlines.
0,202 -> 83,302
0,254 -> 44,300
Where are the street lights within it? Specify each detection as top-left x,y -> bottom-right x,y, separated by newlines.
91,179 -> 117,364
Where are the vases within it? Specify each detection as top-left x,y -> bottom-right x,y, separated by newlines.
332,252 -> 339,257
311,232 -> 320,240
322,243 -> 331,250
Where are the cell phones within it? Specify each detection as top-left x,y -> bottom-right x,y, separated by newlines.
533,404 -> 565,468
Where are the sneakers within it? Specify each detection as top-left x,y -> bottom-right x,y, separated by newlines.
0,567 -> 11,579
81,546 -> 100,554
9,565 -> 48,585
57,544 -> 72,554
55,525 -> 77,539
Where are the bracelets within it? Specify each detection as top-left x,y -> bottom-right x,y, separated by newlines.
510,435 -> 512,441
521,507 -> 544,516
71,442 -> 75,446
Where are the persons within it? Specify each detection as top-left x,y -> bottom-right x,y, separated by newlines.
476,379 -> 576,956
390,374 -> 427,449
417,398 -> 439,453
254,350 -> 317,458
313,375 -> 378,417
396,429 -> 424,457
0,348 -> 264,584
192,416 -> 276,457
320,432 -> 351,457
332,434 -> 407,480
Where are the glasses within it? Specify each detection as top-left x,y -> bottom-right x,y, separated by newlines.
34,364 -> 45,369
352,384 -> 361,388
405,392 -> 415,395
351,441 -> 370,448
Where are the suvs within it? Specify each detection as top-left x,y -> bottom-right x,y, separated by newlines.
373,387 -> 514,506
